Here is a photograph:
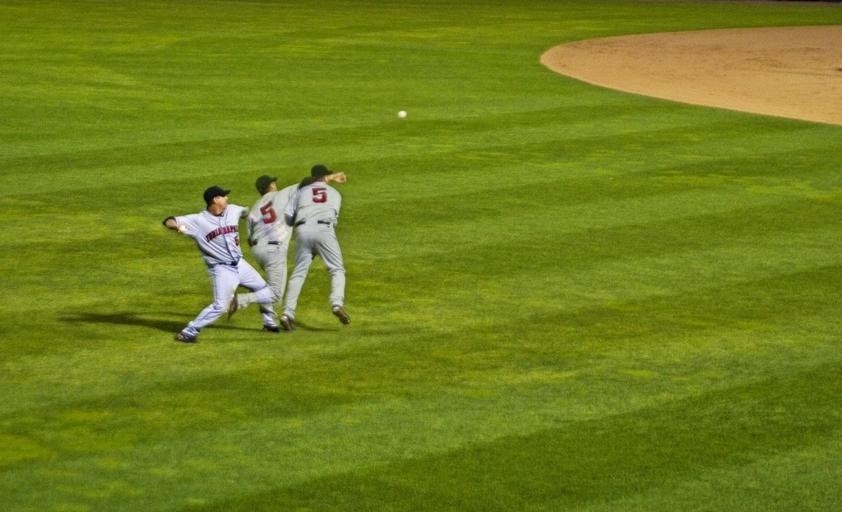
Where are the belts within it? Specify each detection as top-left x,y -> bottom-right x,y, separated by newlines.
251,240 -> 281,245
295,220 -> 331,226
219,261 -> 238,266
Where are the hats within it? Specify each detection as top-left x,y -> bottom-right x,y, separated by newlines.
311,165 -> 332,177
256,175 -> 277,194
203,186 -> 232,204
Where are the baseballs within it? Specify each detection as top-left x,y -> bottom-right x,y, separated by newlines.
397,110 -> 407,119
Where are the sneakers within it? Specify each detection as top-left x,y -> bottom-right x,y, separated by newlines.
174,332 -> 199,343
332,306 -> 350,324
264,325 -> 280,332
228,291 -> 237,317
280,315 -> 295,331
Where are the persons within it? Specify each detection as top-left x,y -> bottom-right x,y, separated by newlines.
277,164 -> 354,331
225,171 -> 351,329
161,184 -> 280,343
